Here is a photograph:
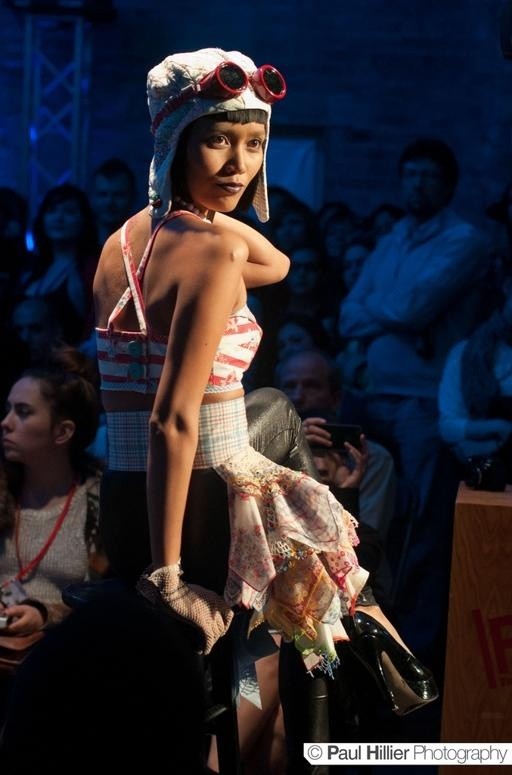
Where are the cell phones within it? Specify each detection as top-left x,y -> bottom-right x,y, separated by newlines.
310,423 -> 362,449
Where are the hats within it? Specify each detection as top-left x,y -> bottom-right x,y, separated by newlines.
147,46 -> 271,225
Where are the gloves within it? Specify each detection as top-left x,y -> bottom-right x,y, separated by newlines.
143,558 -> 233,655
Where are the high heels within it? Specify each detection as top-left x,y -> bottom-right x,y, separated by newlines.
341,610 -> 440,715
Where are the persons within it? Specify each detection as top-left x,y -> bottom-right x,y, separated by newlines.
90,45 -> 439,775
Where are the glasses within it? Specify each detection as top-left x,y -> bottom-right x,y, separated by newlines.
153,60 -> 288,128
